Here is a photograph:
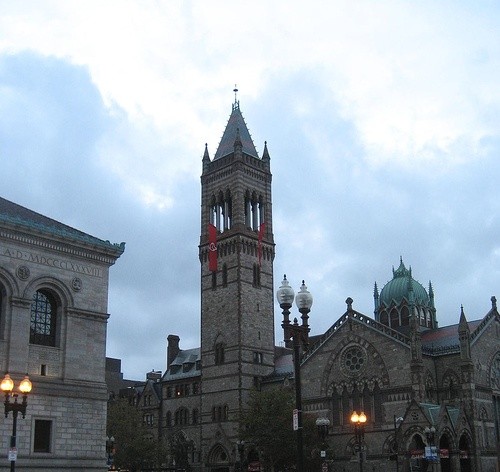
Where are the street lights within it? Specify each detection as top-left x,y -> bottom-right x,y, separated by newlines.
422,426 -> 437,472
104,435 -> 116,464
315,416 -> 330,464
392,414 -> 404,472
351,408 -> 368,472
236,440 -> 246,472
277,274 -> 314,472
0,372 -> 32,472
